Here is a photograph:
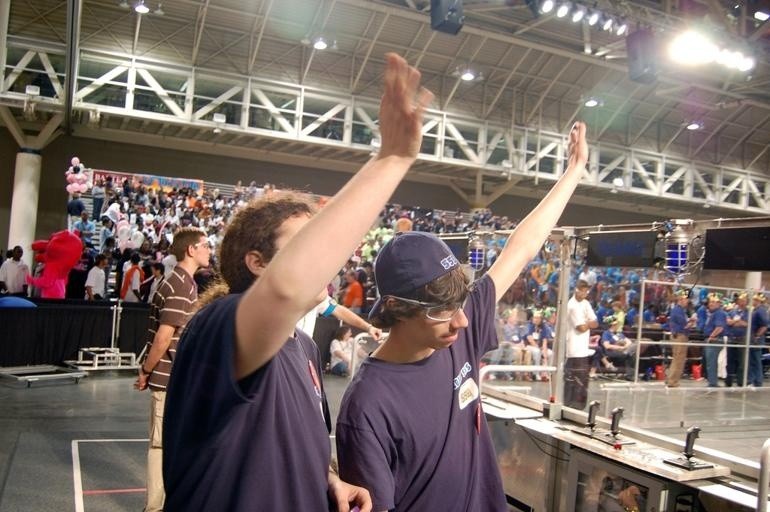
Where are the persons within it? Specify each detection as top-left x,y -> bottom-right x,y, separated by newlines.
133,226 -> 213,510
566,279 -> 600,411
1,176 -> 769,389
159,49 -> 435,512
335,120 -> 590,511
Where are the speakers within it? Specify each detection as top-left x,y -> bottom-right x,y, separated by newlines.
430,1 -> 465,36
625,29 -> 659,83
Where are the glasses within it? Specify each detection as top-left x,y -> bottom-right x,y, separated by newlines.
382,294 -> 468,321
195,242 -> 209,250
575,287 -> 590,296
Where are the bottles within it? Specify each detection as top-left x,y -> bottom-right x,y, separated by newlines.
325,362 -> 331,374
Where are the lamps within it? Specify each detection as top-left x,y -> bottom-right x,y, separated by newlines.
585,97 -> 598,109
538,1 -> 628,40
454,64 -> 483,83
300,30 -> 337,51
118,1 -> 167,16
686,121 -> 700,131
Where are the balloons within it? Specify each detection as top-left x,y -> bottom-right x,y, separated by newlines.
64,156 -> 92,195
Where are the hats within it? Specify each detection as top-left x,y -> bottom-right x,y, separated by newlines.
531,308 -> 543,317
675,291 -> 689,300
708,293 -> 724,302
367,231 -> 460,327
575,280 -> 592,288
754,293 -> 766,302
603,316 -> 619,326
735,291 -> 749,298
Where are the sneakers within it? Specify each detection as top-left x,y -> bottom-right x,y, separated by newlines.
605,363 -> 619,373
523,374 -> 533,382
533,375 -> 542,381
541,377 -> 549,381
505,374 -> 514,381
588,373 -> 598,381
515,375 -> 521,382
